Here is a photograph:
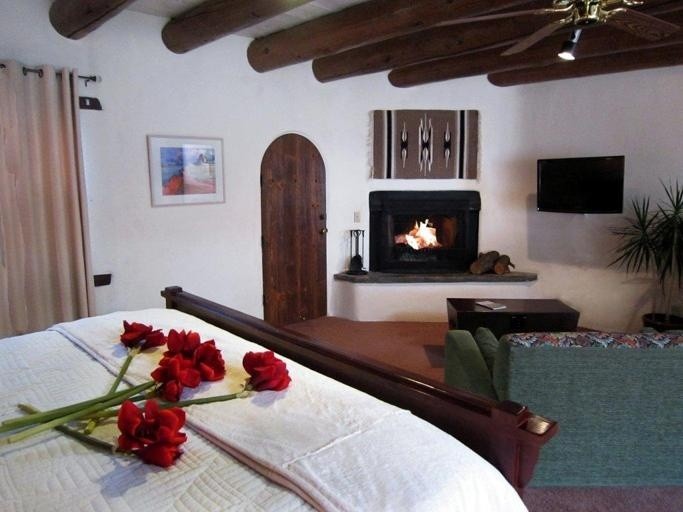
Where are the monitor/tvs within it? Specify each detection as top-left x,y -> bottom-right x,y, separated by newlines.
536,155 -> 625,214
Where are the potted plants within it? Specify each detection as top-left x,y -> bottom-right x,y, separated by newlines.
604,179 -> 683,331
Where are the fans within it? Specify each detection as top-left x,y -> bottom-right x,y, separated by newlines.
431,0 -> 680,58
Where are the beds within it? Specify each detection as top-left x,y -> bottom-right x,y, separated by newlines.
0,282 -> 560,511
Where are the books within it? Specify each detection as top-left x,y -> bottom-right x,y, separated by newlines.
475,299 -> 507,311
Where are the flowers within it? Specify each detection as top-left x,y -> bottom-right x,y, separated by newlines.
0,314 -> 290,469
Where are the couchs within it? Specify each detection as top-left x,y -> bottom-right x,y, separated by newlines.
443,323 -> 683,488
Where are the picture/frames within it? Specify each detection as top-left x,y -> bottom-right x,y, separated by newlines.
146,133 -> 225,207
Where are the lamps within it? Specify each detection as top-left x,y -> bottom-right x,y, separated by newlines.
556,27 -> 586,63
